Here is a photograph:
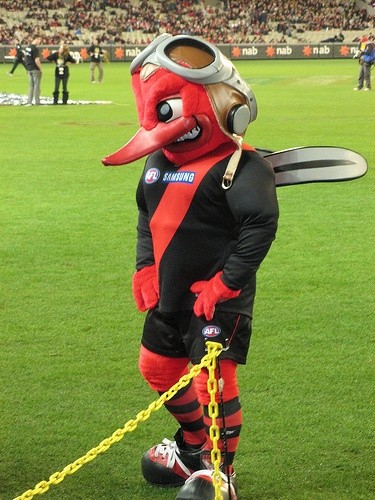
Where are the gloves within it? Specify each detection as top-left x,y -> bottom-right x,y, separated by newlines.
190,270 -> 241,321
133,263 -> 160,313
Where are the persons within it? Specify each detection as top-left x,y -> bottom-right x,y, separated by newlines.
47,44 -> 76,104
354,33 -> 375,92
7,43 -> 28,76
0,0 -> 375,46
102,34 -> 279,500
22,34 -> 42,107
87,40 -> 104,84
